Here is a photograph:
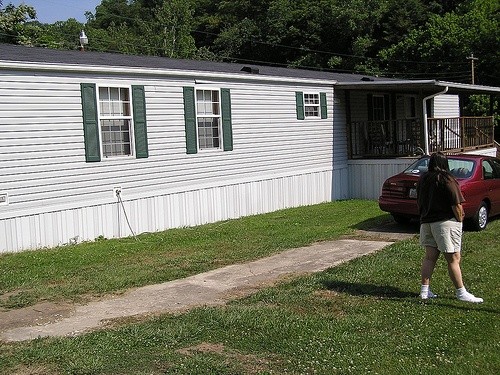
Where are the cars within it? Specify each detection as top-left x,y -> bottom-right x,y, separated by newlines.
378,154 -> 500,231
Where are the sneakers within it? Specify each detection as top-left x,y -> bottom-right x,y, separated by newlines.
456,286 -> 484,303
420,289 -> 438,300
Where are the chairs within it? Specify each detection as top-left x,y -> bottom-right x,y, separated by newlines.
395,119 -> 422,157
363,121 -> 394,156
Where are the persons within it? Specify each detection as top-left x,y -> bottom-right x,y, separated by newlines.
417,151 -> 483,303
